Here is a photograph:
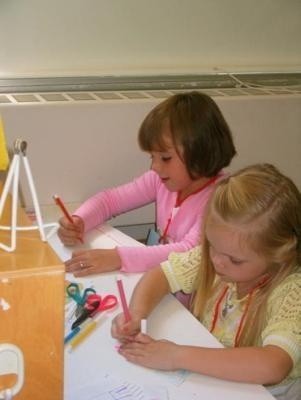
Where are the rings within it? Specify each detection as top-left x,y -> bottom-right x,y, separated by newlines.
79,261 -> 84,268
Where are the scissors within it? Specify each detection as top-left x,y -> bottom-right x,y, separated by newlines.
65,282 -> 118,330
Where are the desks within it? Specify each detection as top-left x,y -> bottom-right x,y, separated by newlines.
43,222 -> 276,400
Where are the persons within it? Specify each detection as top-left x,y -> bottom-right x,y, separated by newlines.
109,162 -> 301,400
56,90 -> 239,313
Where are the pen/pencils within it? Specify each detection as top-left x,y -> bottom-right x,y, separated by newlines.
64,310 -> 107,350
116,274 -> 137,343
53,195 -> 85,245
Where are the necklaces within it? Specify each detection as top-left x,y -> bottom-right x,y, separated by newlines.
221,284 -> 261,317
